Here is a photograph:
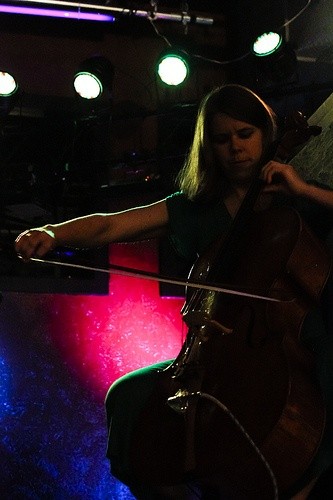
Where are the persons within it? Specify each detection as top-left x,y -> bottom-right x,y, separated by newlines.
14,84 -> 333,500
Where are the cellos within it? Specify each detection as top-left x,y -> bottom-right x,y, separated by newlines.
127,111 -> 333,500
